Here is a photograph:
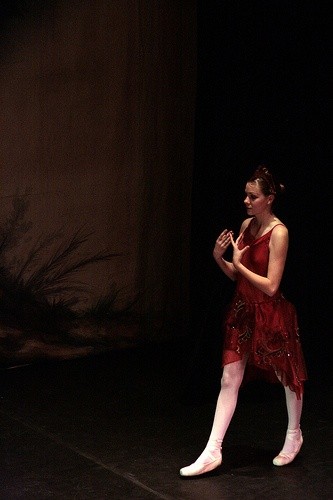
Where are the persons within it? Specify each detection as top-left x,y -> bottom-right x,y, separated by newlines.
176,166 -> 306,480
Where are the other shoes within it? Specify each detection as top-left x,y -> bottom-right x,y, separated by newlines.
273,435 -> 303,466
180,452 -> 222,476
